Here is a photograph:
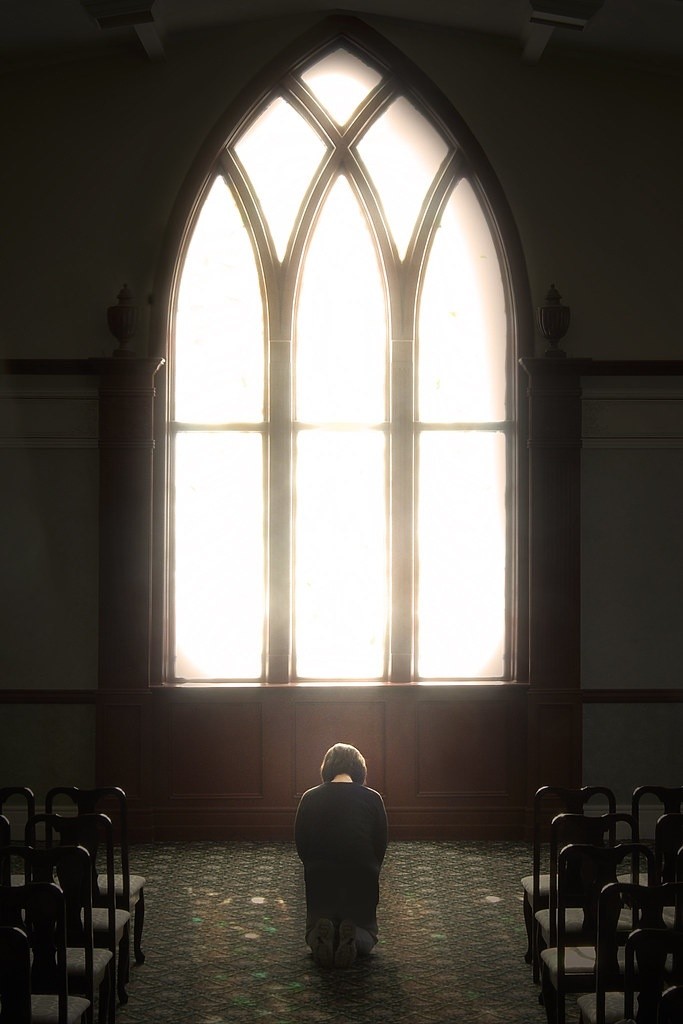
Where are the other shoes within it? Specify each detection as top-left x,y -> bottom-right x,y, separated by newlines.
334,919 -> 356,970
312,919 -> 334,971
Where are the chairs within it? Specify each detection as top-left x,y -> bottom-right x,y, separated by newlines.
0,784 -> 144,1024
521,780 -> 683,1024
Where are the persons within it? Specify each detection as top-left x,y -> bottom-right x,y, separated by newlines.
295,742 -> 389,971
